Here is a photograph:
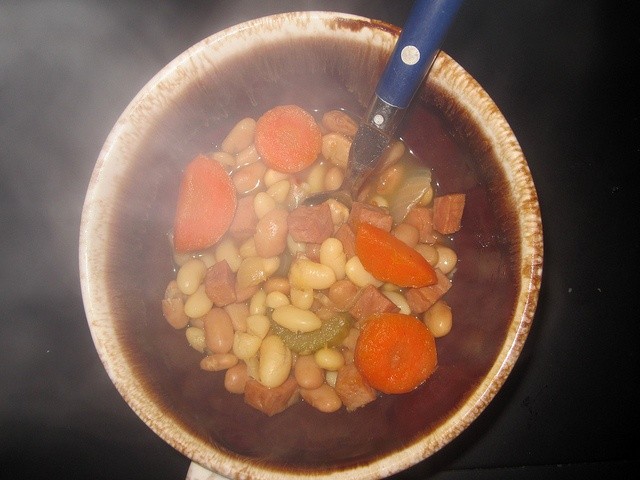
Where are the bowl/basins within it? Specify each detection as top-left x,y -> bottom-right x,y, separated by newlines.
80,10 -> 545,476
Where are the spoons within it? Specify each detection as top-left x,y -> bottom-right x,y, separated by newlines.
298,0 -> 461,210
